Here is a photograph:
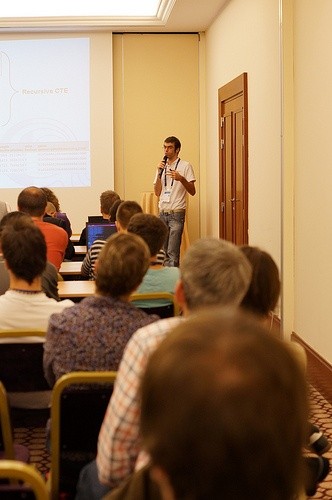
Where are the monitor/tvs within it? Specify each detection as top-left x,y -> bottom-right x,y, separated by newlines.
86,222 -> 116,251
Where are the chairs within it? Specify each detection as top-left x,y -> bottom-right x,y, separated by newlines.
0,291 -> 178,500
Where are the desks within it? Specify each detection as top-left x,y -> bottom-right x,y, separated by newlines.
59,262 -> 83,275
74,246 -> 87,254
57,281 -> 96,297
70,235 -> 80,242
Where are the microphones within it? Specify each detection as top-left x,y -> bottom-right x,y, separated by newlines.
159,156 -> 167,175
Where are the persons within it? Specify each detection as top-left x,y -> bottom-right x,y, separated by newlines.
0,215 -> 81,427
77,237 -> 254,500
233,245 -> 331,457
154,136 -> 196,267
45,230 -> 162,456
139,306 -> 308,500
0,187 -> 186,310
